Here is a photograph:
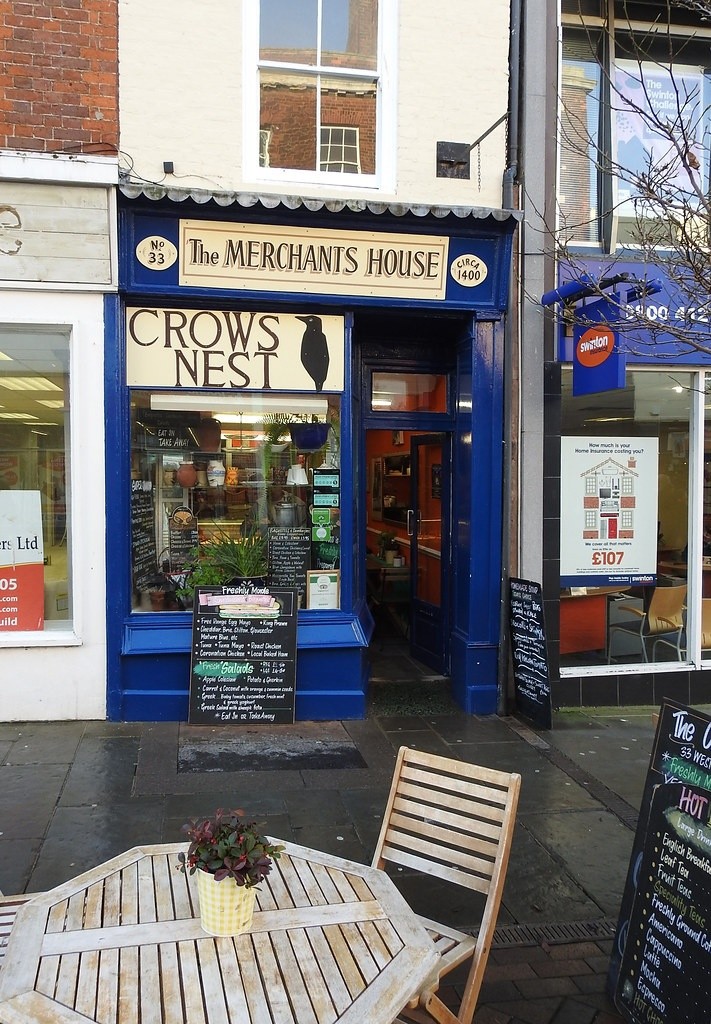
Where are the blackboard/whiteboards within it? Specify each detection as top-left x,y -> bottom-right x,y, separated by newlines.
506,577 -> 553,732
189,582 -> 298,725
603,695 -> 711,1024
129,479 -> 201,592
264,526 -> 313,609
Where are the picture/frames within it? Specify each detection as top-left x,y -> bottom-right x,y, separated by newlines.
392,431 -> 404,445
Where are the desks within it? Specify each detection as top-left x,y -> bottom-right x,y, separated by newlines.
366,553 -> 410,638
560,546 -> 711,665
0,834 -> 442,1024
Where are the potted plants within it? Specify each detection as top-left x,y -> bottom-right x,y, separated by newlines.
175,809 -> 286,939
260,408 -> 341,488
376,529 -> 400,564
142,514 -> 303,611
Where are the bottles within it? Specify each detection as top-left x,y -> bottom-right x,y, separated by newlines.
384,543 -> 406,567
199,416 -> 221,452
385,495 -> 397,507
164,460 -> 239,487
275,492 -> 297,527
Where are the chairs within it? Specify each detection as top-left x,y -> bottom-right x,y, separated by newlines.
651,598 -> 711,663
368,568 -> 423,651
0,746 -> 523,1024
607,584 -> 688,664
